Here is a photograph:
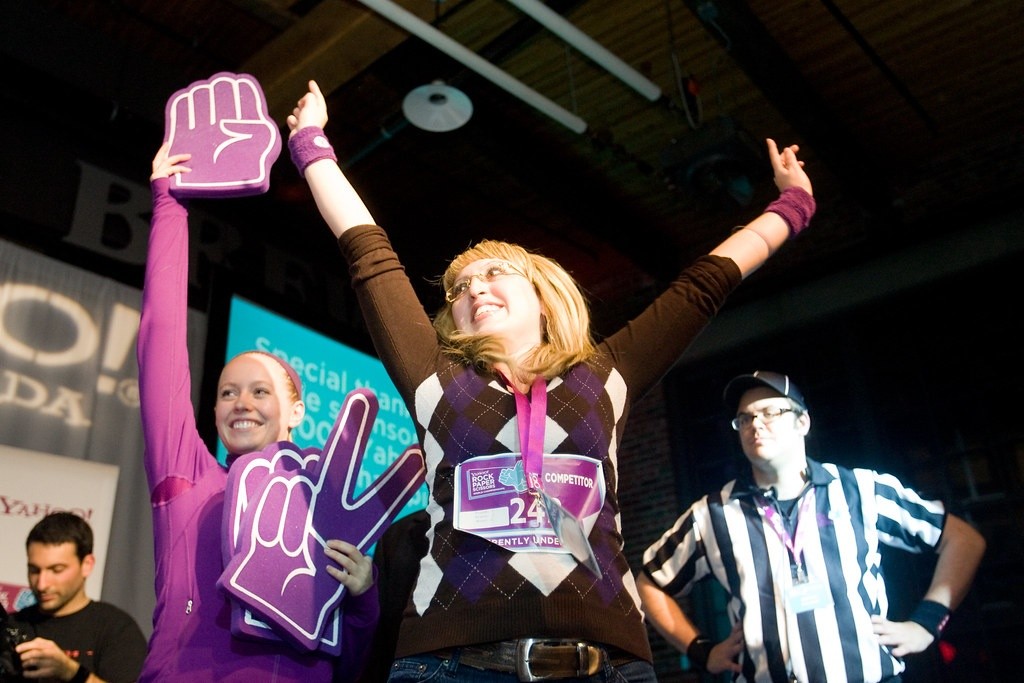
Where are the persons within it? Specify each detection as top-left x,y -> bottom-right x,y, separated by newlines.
635,371 -> 988,683
284,79 -> 816,683
133,137 -> 381,683
0,508 -> 150,683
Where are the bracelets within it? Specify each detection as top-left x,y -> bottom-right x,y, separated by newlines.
289,125 -> 338,179
686,635 -> 718,675
763,187 -> 817,238
909,599 -> 952,640
67,663 -> 91,683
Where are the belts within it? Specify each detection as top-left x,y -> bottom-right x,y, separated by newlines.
426,637 -> 644,683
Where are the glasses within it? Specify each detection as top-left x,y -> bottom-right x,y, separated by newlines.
732,406 -> 803,431
445,261 -> 538,303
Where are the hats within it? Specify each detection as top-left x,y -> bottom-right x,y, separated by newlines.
724,371 -> 807,420
